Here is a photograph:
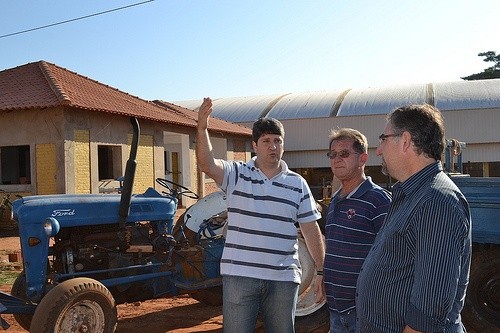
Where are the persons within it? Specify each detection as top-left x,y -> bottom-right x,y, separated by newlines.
356,104 -> 472,333
322,127 -> 392,333
197,97 -> 325,333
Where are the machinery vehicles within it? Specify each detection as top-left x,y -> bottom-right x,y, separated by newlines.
0,114 -> 500,333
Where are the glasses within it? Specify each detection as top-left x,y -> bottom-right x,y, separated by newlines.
327,150 -> 360,159
379,133 -> 414,144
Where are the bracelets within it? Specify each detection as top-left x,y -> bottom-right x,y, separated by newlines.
317,270 -> 323,275
